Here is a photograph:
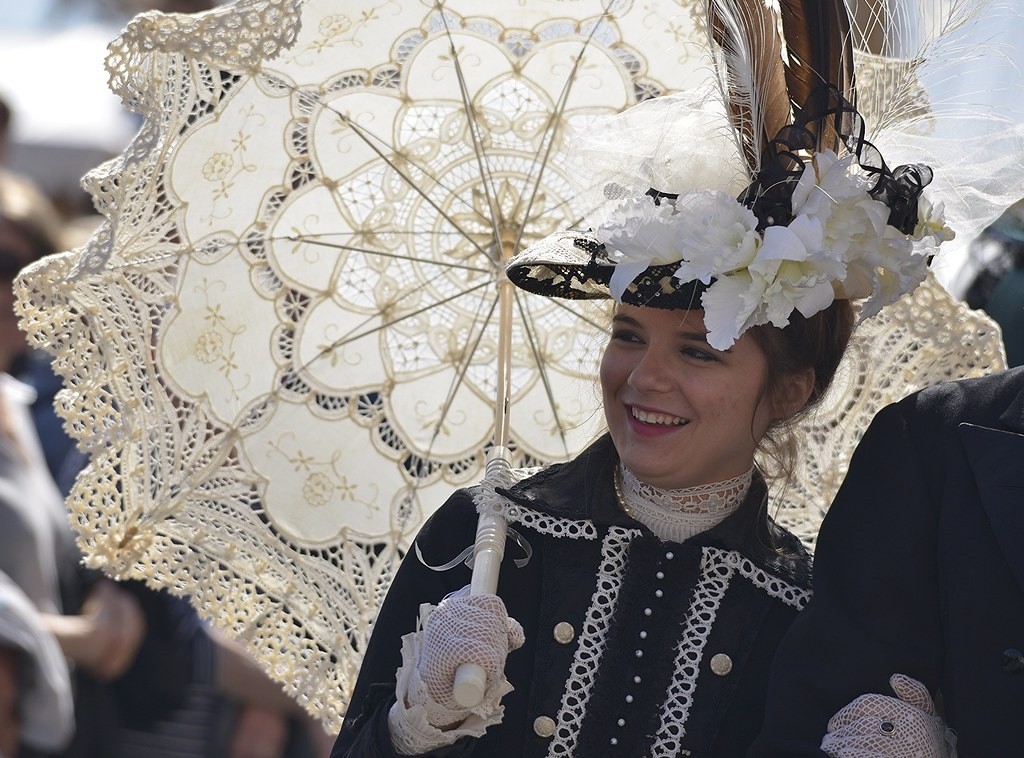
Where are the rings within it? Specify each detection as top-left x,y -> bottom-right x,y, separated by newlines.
877,716 -> 896,738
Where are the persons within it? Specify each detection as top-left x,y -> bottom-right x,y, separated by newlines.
812,362 -> 1024,758
327,1 -> 967,758
0,99 -> 322,758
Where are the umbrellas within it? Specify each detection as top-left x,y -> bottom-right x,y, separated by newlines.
14,0 -> 1010,732
